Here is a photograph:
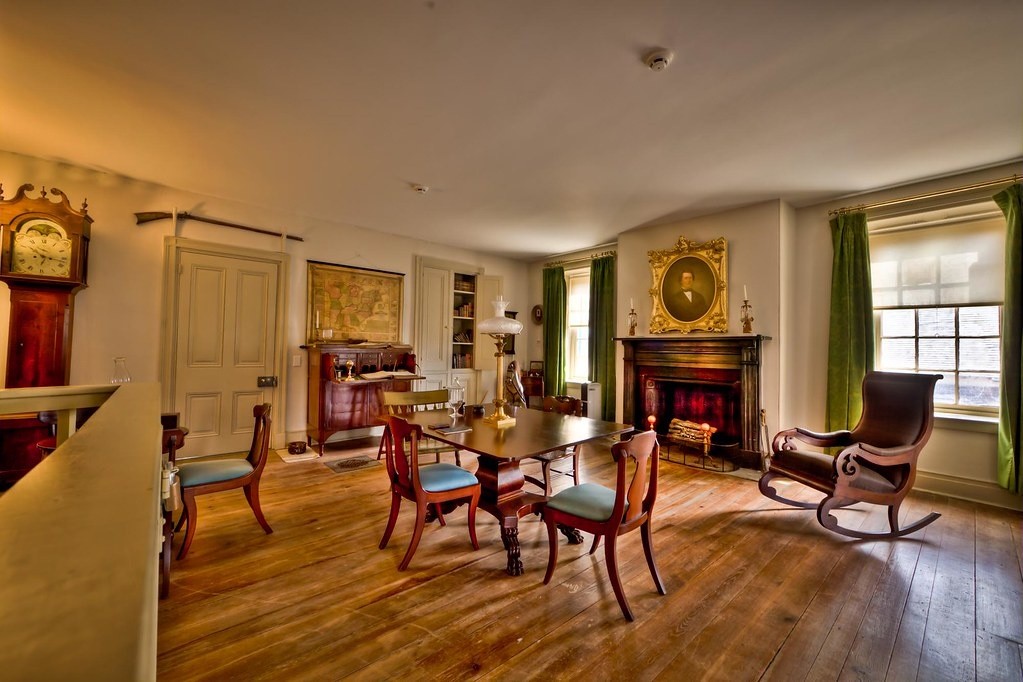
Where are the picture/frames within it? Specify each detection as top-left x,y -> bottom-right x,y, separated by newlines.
304,259 -> 404,348
647,232 -> 731,334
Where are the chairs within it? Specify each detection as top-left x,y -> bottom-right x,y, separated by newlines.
757,371 -> 946,540
379,415 -> 482,572
521,394 -> 584,496
538,428 -> 670,623
383,388 -> 462,471
170,400 -> 273,561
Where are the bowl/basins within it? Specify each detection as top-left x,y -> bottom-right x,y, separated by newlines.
287,441 -> 307,454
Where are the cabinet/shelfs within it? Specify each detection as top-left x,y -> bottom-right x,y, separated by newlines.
300,343 -> 427,457
416,255 -> 503,413
518,375 -> 544,401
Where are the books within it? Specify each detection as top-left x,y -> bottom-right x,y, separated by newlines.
454,331 -> 470,342
436,427 -> 473,435
454,302 -> 472,317
455,280 -> 474,292
428,423 -> 449,429
453,353 -> 470,369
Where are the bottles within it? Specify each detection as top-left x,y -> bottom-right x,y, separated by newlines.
109,356 -> 132,384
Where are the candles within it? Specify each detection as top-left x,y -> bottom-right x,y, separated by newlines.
744,284 -> 748,299
316,310 -> 320,328
630,297 -> 634,309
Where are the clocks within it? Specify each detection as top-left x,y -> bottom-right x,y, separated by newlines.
0,181 -> 94,492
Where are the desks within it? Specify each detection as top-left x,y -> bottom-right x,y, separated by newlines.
35,426 -> 189,564
397,399 -> 634,574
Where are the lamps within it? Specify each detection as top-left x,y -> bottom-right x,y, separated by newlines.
476,292 -> 524,402
